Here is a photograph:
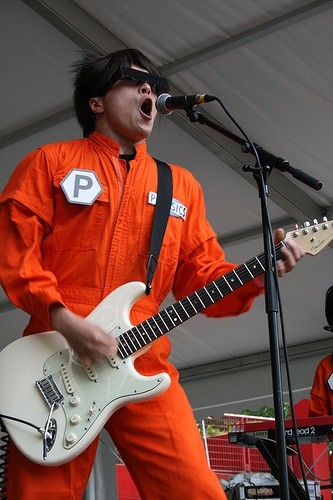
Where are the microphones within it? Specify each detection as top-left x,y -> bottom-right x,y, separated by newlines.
155,93 -> 214,116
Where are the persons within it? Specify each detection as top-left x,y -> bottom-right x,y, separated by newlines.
0,49 -> 305,500
308,353 -> 333,418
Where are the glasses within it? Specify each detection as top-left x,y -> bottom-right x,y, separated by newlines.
99,65 -> 170,97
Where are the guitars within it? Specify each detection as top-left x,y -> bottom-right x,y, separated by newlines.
0,217 -> 333,466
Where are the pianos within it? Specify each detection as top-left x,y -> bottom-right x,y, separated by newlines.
228,416 -> 332,446
